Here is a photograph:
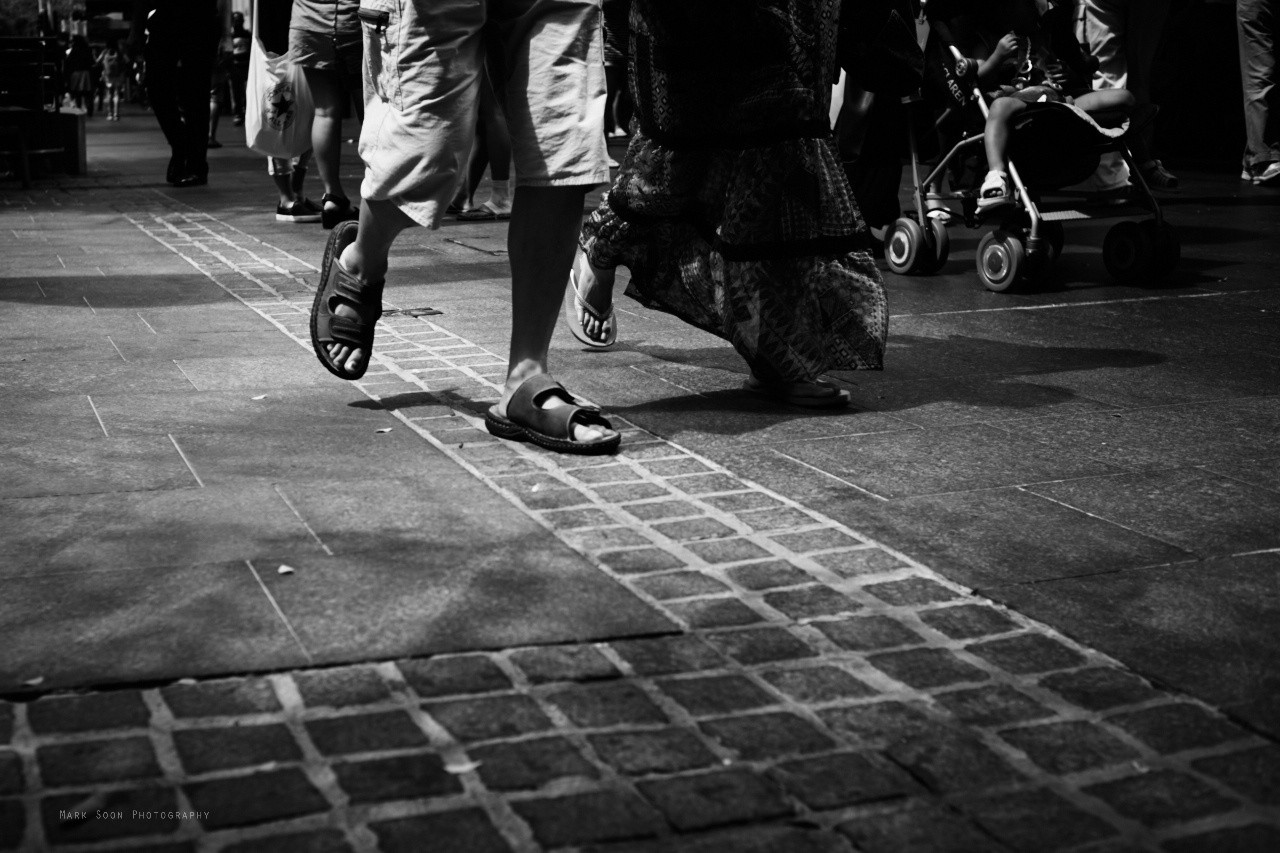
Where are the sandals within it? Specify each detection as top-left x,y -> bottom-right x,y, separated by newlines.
977,171 -> 1009,205
1128,158 -> 1181,198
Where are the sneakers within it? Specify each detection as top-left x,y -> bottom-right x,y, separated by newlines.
275,196 -> 323,222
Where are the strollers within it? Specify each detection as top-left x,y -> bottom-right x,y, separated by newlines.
884,0 -> 1182,294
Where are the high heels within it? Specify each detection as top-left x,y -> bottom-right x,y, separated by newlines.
319,193 -> 359,229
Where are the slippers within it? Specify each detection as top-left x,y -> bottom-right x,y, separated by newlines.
309,221 -> 385,381
910,195 -> 951,225
488,373 -> 621,454
741,377 -> 850,404
563,244 -> 617,347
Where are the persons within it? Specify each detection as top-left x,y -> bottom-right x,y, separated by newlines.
832,0 -> 1280,227
607,152 -> 621,169
0,0 -> 511,225
563,0 -> 892,407
307,0 -> 627,454
599,0 -> 629,138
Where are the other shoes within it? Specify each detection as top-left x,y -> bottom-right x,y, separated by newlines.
1078,182 -> 1132,210
440,195 -> 475,217
465,200 -> 514,219
207,137 -> 223,149
1241,161 -> 1280,181
167,148 -> 208,187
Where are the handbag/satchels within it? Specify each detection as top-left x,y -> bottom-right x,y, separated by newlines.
244,37 -> 315,158
